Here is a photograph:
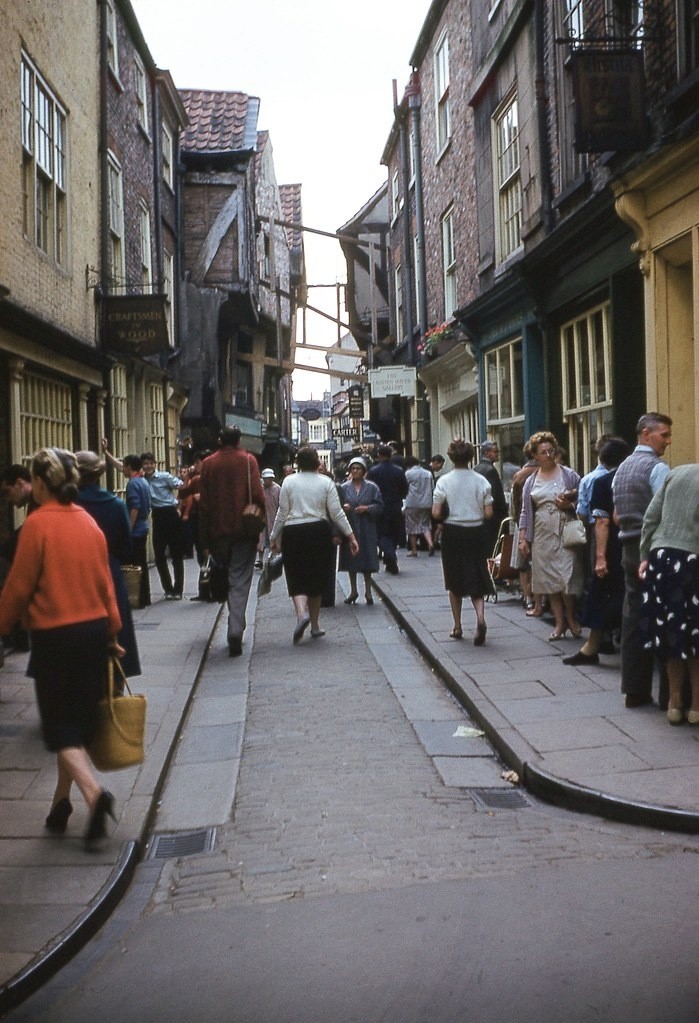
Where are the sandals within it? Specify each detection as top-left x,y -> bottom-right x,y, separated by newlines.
474,623 -> 486,645
451,627 -> 462,638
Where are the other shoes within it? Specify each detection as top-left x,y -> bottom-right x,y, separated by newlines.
407,543 -> 434,557
562,642 -> 615,666
183,552 -> 195,559
229,641 -> 242,657
255,561 -> 264,569
522,594 -> 548,616
164,588 -> 201,601
624,689 -> 699,725
292,616 -> 310,642
386,562 -> 400,574
311,627 -> 325,636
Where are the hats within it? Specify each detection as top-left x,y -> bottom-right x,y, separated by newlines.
261,468 -> 275,478
349,456 -> 368,474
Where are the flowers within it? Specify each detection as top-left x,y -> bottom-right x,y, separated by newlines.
417,321 -> 455,355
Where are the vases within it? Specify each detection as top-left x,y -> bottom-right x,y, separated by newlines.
437,338 -> 458,356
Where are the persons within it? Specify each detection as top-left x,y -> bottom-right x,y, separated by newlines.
610,413 -> 674,710
575,434 -> 618,653
431,439 -> 495,644
101,437 -> 193,601
519,431 -> 585,641
0,465 -> 41,559
196,425 -> 266,657
179,450 -> 216,603
319,457 -> 386,608
268,448 -> 359,645
75,452 -> 141,696
121,455 -> 152,608
473,441 -> 509,586
561,439 -> 631,666
0,447 -> 124,851
255,465 -> 293,569
638,464 -> 699,729
511,440 -> 549,609
363,440 -> 445,575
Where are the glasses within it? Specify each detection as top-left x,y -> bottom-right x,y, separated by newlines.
533,447 -> 556,456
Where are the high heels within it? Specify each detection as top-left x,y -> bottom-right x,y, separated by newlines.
345,593 -> 359,604
87,791 -> 121,844
365,594 -> 374,605
45,798 -> 73,831
550,616 -> 582,641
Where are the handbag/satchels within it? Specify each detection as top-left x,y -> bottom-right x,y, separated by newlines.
199,554 -> 227,603
561,504 -> 589,547
243,504 -> 266,538
120,555 -> 142,608
83,653 -> 146,773
257,544 -> 284,599
